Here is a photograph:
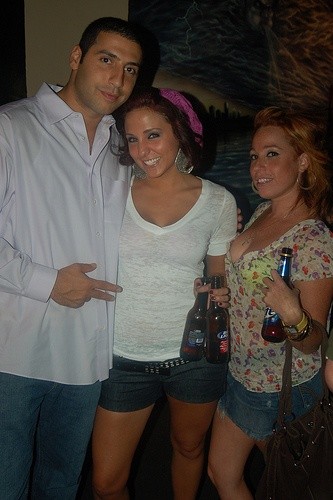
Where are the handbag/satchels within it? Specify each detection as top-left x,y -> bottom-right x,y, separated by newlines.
251,320 -> 333,500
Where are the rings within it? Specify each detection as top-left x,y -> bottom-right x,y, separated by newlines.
80,303 -> 84,307
86,296 -> 91,302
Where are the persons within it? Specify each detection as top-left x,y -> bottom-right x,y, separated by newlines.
323,328 -> 333,393
92,90 -> 238,500
0,16 -> 243,500
207,107 -> 333,500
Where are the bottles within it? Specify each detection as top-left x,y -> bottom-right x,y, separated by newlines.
207,276 -> 229,364
261,248 -> 293,343
179,278 -> 210,362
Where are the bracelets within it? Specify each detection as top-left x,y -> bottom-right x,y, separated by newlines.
282,319 -> 313,343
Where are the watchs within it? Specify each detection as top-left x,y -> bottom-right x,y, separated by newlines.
281,313 -> 308,334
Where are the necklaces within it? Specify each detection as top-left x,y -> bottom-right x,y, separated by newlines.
241,202 -> 305,246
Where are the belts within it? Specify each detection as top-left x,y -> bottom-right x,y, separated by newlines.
113,358 -> 189,376
